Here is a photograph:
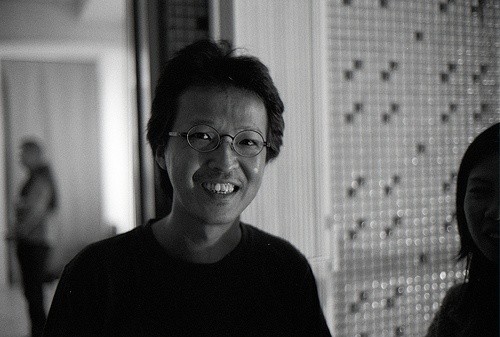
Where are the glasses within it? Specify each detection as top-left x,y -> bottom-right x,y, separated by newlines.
167,124 -> 271,158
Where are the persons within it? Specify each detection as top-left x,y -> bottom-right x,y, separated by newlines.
45,39 -> 332,337
4,141 -> 51,337
422,123 -> 500,336
